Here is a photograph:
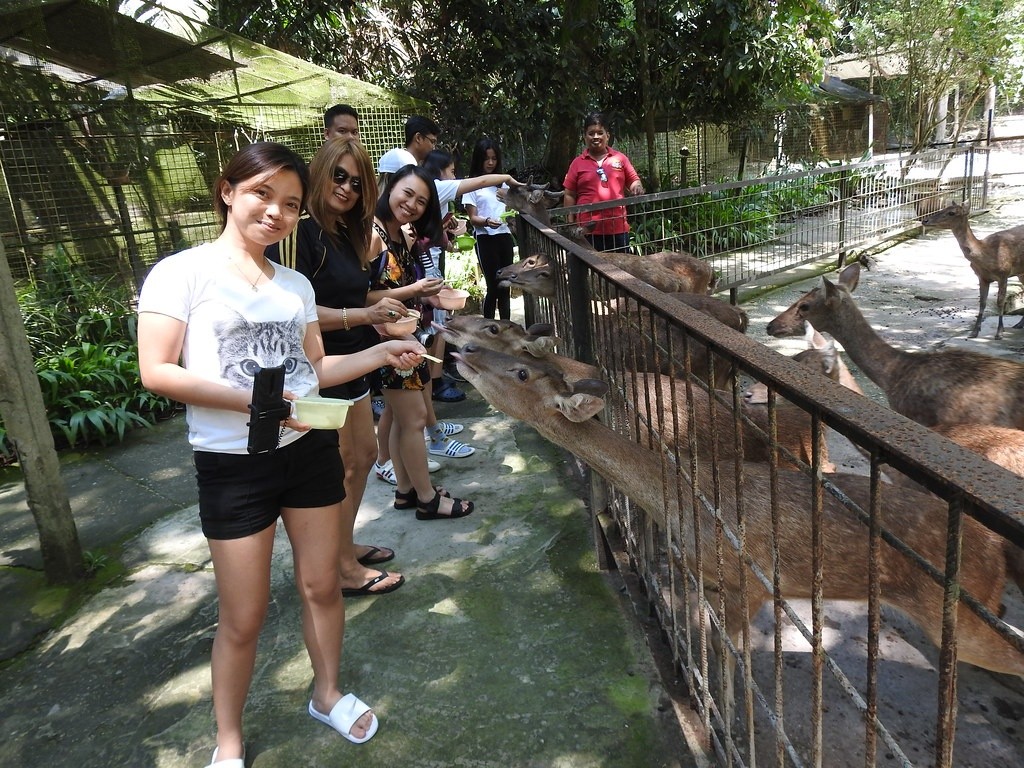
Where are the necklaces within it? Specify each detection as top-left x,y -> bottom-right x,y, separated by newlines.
213,242 -> 265,293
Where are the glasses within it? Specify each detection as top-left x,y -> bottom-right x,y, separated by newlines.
419,131 -> 437,149
330,165 -> 362,194
598,167 -> 607,181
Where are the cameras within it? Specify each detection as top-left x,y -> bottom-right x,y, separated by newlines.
412,326 -> 435,348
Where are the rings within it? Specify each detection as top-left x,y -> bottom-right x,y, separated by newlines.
388,311 -> 396,318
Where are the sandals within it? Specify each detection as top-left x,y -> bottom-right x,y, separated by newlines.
393,486 -> 451,509
443,363 -> 466,382
416,493 -> 474,519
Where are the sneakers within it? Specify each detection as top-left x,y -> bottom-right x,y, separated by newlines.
427,458 -> 441,473
375,459 -> 398,485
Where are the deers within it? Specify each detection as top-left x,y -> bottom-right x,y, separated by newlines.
427,198 -> 1024,743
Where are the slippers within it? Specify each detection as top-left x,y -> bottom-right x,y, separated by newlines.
309,693 -> 378,744
430,440 -> 475,457
432,384 -> 465,402
358,545 -> 394,564
205,741 -> 246,768
342,569 -> 405,597
424,422 -> 463,441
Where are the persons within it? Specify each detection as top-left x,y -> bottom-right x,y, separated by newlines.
563,113 -> 645,253
137,104 -> 527,768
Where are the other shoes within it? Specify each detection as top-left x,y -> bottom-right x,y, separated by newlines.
372,400 -> 385,420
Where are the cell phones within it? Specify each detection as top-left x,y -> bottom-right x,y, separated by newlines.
442,212 -> 454,224
247,364 -> 286,455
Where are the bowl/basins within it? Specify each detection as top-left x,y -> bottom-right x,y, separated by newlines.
384,309 -> 420,336
457,237 -> 476,251
292,398 -> 354,429
449,219 -> 467,235
437,289 -> 470,310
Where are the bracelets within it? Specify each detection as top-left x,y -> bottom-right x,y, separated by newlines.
342,307 -> 351,331
486,217 -> 491,227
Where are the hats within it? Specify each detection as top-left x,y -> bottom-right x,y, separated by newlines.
377,148 -> 418,173
